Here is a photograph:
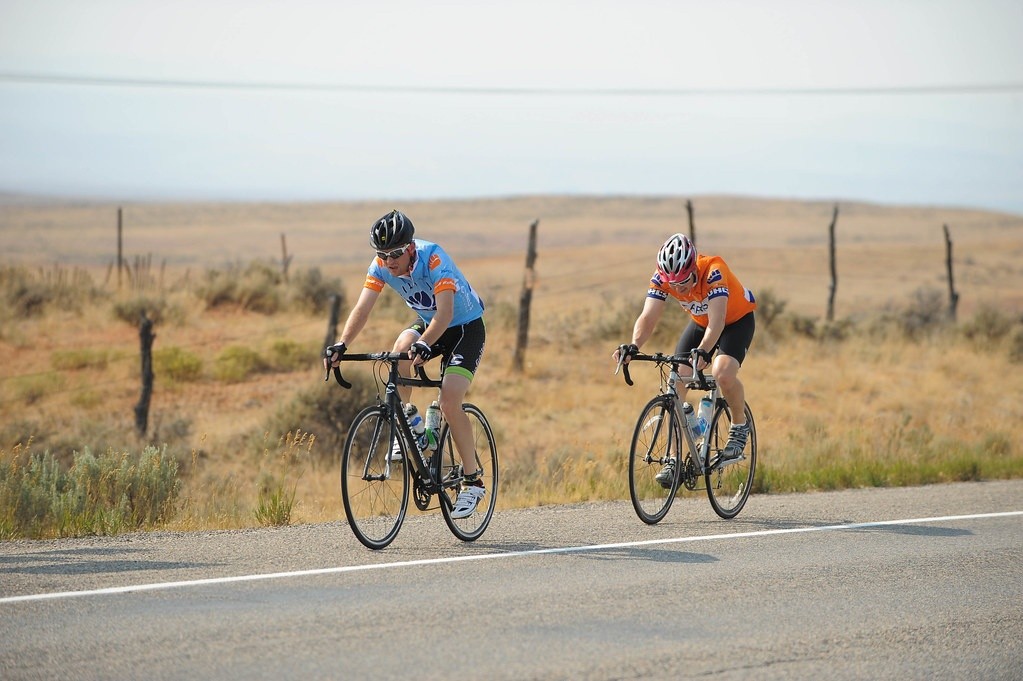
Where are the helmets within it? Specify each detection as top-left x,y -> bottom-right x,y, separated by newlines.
657,233 -> 697,282
369,210 -> 414,250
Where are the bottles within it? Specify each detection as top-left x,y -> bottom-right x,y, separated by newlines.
698,395 -> 713,437
424,401 -> 441,450
683,402 -> 701,437
406,403 -> 428,449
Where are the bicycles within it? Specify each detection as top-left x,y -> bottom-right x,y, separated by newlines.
320,342 -> 500,551
615,343 -> 758,526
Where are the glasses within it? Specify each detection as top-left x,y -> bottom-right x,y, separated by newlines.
669,273 -> 692,286
376,244 -> 410,260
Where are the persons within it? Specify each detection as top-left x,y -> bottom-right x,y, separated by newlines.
612,233 -> 757,483
323,210 -> 486,520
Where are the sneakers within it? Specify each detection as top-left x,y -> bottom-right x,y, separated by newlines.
450,478 -> 486,519
723,418 -> 750,458
655,458 -> 677,485
385,436 -> 403,461
